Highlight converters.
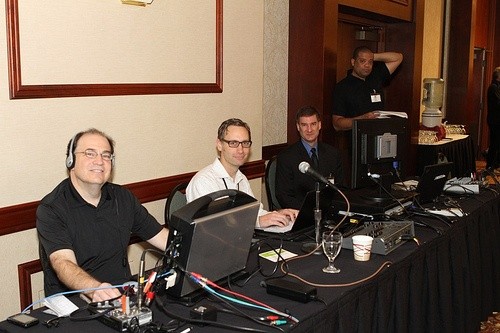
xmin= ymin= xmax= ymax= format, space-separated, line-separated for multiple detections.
xmin=266 ymin=279 xmax=318 ymax=304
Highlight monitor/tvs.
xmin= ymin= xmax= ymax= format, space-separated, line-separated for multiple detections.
xmin=351 ymin=118 xmax=407 ymax=202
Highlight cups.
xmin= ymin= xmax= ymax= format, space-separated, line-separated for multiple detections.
xmin=352 ymin=235 xmax=374 ymax=262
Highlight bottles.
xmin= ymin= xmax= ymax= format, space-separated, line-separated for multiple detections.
xmin=421 ymin=78 xmax=444 ymax=109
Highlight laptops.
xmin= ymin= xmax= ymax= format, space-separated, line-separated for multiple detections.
xmin=412 ymin=161 xmax=454 ymax=206
xmin=254 ymin=184 xmax=340 ymax=236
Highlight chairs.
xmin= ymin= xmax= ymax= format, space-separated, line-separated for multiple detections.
xmin=17 ymin=258 xmax=45 ymax=313
xmin=165 ymin=182 xmax=191 ymax=235
xmin=265 ymin=156 xmax=288 ymax=211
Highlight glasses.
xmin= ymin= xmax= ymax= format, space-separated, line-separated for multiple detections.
xmin=75 ymin=149 xmax=115 ymax=161
xmin=221 ymin=139 xmax=252 ymax=149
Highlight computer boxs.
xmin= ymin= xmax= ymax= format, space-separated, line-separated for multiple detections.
xmin=330 ymin=189 xmax=421 ymax=223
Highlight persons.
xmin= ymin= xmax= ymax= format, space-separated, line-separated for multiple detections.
xmin=36 ymin=128 xmax=169 ymax=304
xmin=185 ymin=117 xmax=299 ymax=228
xmin=487 ymin=67 xmax=500 ymax=170
xmin=275 ymin=106 xmax=354 ymax=225
xmin=331 ymin=47 xmax=403 ymax=184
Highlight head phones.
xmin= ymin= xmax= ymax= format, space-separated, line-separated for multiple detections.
xmin=66 ymin=136 xmax=77 ymax=169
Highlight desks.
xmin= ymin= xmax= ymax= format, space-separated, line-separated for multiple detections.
xmin=0 ymin=177 xmax=500 ymax=333
xmin=418 ymin=129 xmax=477 ymax=178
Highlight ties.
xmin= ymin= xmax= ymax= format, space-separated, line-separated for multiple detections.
xmin=310 ymin=148 xmax=320 ymax=174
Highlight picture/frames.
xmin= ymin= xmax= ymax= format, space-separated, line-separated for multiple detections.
xmin=5 ymin=0 xmax=225 ymax=100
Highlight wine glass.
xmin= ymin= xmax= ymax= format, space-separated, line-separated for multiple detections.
xmin=322 ymin=231 xmax=343 ymax=274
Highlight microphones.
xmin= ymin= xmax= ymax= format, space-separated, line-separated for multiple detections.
xmin=299 ymin=162 xmax=338 ymax=190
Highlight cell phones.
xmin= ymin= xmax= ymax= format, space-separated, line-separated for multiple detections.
xmin=7 ymin=313 xmax=39 ymax=328
